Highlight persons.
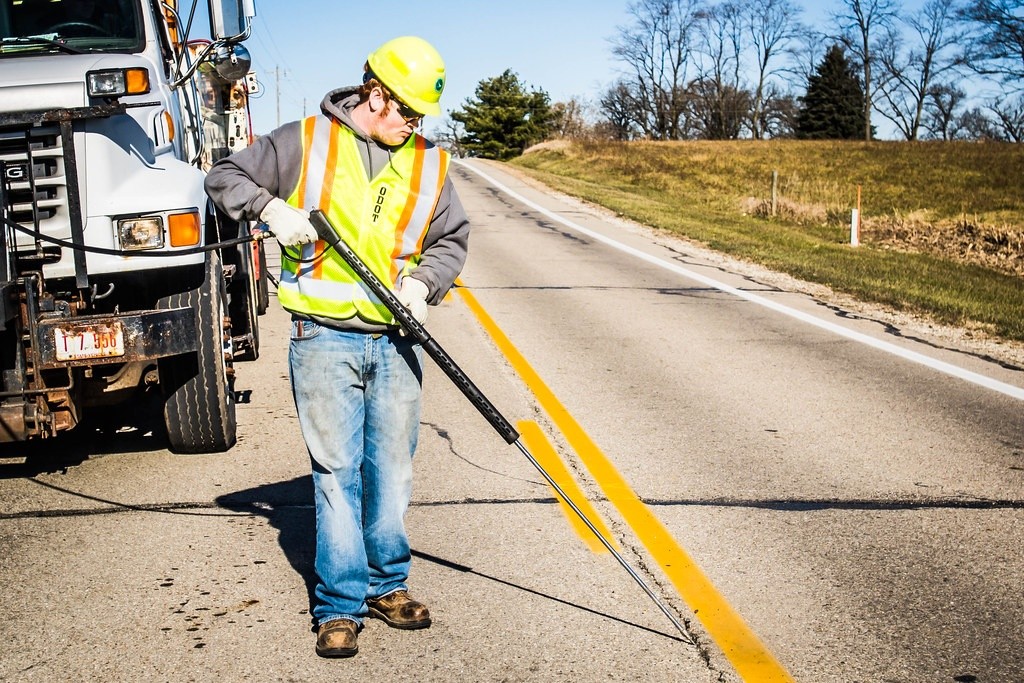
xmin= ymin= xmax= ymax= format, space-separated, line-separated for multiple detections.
xmin=200 ymin=34 xmax=477 ymax=660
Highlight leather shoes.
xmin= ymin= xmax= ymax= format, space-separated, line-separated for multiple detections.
xmin=315 ymin=619 xmax=360 ymax=658
xmin=366 ymin=591 xmax=432 ymax=629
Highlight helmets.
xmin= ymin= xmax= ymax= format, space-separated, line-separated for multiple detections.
xmin=366 ymin=36 xmax=446 ymax=115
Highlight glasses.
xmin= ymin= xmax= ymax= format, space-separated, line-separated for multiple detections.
xmin=388 ymin=93 xmax=426 ymax=123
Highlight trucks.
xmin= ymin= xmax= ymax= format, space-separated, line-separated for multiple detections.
xmin=1 ymin=0 xmax=271 ymax=453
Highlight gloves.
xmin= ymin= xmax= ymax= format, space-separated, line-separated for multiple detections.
xmin=389 ymin=279 xmax=430 ymax=337
xmin=259 ymin=196 xmax=319 ymax=247
